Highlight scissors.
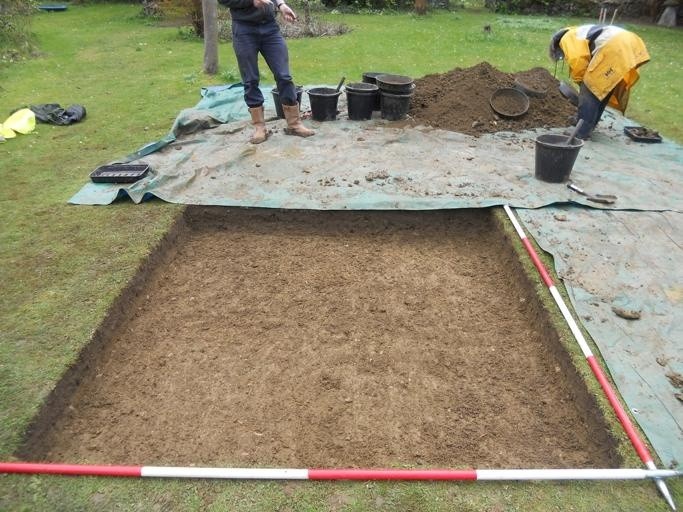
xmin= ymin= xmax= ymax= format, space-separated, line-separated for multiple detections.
xmin=567 ymin=183 xmax=617 ymax=203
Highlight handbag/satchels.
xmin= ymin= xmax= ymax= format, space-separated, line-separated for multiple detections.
xmin=277 ymin=3 xmax=286 ymax=8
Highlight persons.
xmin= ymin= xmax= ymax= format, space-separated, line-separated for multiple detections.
xmin=218 ymin=0 xmax=316 ymax=144
xmin=548 ymin=25 xmax=650 ymax=140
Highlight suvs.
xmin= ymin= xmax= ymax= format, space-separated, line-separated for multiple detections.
xmin=535 ymin=134 xmax=585 ymax=183
xmin=376 ymin=73 xmax=417 ymax=119
xmin=363 ymin=68 xmax=383 ymax=82
xmin=308 ymin=86 xmax=342 ymax=119
xmin=347 ymin=81 xmax=377 ymax=94
xmin=345 ymin=91 xmax=378 ymax=119
xmin=272 ymin=85 xmax=302 ymax=118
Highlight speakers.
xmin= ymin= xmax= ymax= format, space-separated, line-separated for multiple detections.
xmin=248 ymin=107 xmax=267 ymax=143
xmin=282 ymin=102 xmax=314 ymax=136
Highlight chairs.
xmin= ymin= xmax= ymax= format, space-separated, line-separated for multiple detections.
xmin=563 ymin=126 xmax=593 ymax=141
